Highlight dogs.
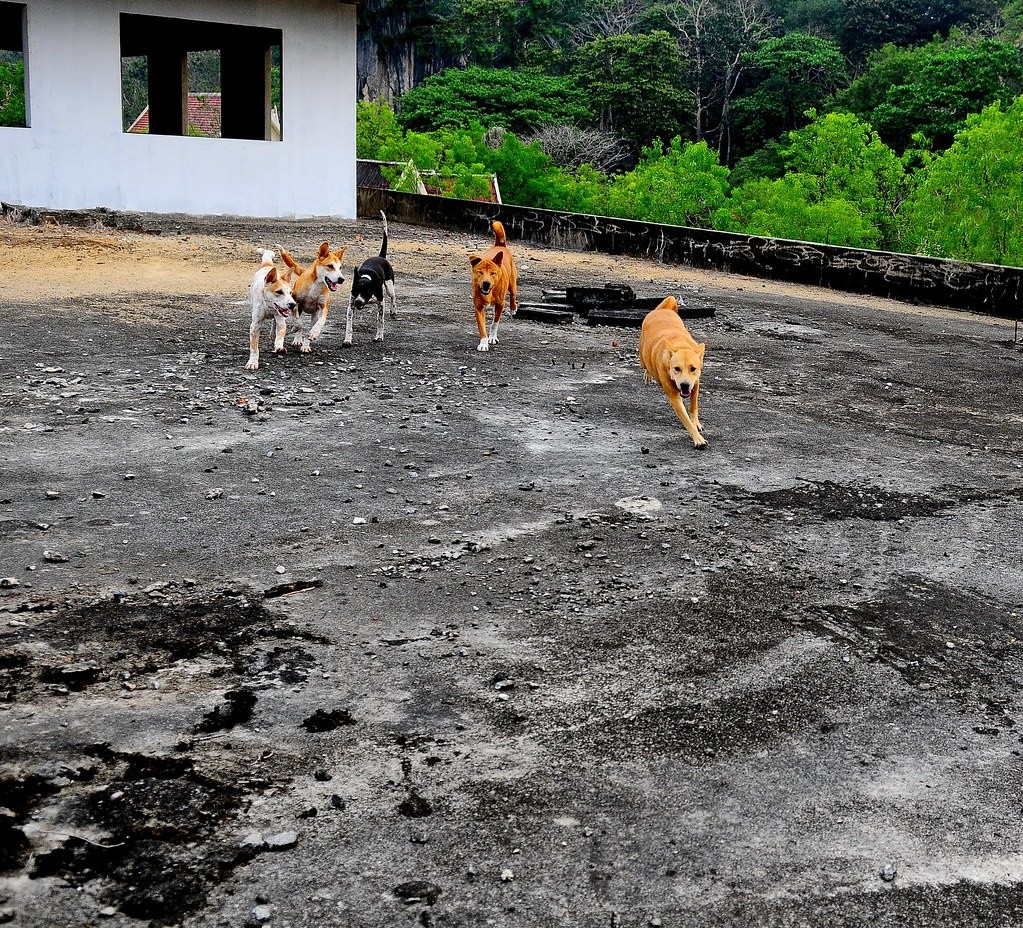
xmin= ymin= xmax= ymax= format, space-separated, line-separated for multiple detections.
xmin=640 ymin=296 xmax=709 ymax=449
xmin=244 ymin=210 xmax=396 ymax=370
xmin=469 ymin=221 xmax=518 ymax=352
